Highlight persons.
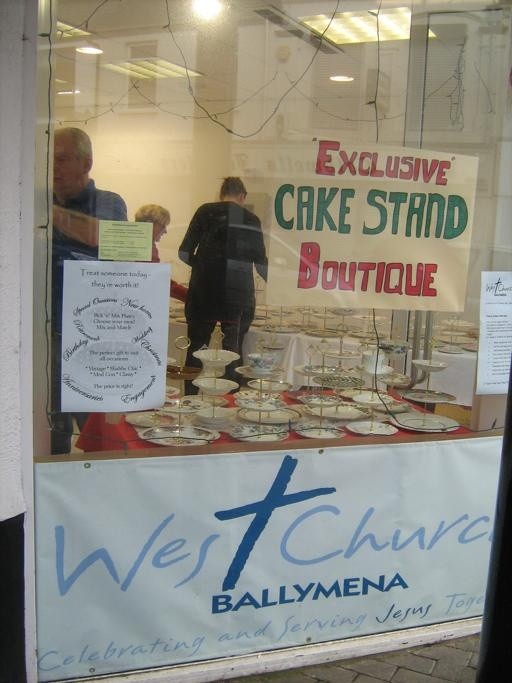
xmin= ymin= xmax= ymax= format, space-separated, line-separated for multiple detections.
xmin=133 ymin=204 xmax=188 ymax=304
xmin=177 ymin=178 xmax=268 ymax=400
xmin=52 ymin=126 xmax=130 ymax=455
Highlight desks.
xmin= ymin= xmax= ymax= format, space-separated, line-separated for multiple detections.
xmin=77 ymin=386 xmax=472 ymax=460
xmin=167 ymin=297 xmax=482 ymax=409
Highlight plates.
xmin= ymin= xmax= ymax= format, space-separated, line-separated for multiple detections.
xmin=125 ymin=289 xmax=478 ymax=446
